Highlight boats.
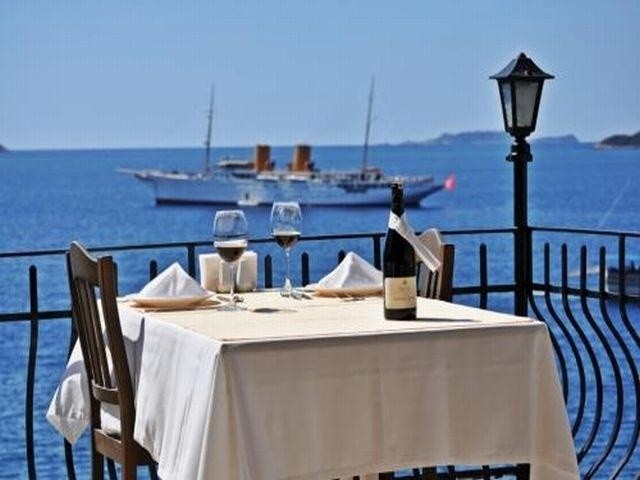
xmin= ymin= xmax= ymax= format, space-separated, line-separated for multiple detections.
xmin=606 ymin=267 xmax=638 ymax=296
xmin=114 ymin=76 xmax=454 ymax=210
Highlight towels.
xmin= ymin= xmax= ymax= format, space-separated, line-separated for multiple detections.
xmin=136 ymin=263 xmax=211 ymax=298
xmin=312 ymin=253 xmax=385 ymax=288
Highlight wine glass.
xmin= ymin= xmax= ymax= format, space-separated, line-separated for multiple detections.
xmin=268 ymin=199 xmax=304 ymax=294
xmin=211 ymin=210 xmax=250 ymax=313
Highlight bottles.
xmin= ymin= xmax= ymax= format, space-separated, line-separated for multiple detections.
xmin=381 ymin=181 xmax=419 ymax=320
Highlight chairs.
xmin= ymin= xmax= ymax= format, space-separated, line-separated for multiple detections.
xmin=415 ymin=229 xmax=454 ymax=304
xmin=66 ymin=242 xmax=160 ymax=480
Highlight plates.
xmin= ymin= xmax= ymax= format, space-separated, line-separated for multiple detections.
xmin=129 ymin=294 xmax=209 ymax=310
xmin=312 ymin=283 xmax=383 ymax=298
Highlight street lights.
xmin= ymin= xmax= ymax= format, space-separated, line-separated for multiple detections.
xmin=486 ymin=50 xmax=555 ymax=316
